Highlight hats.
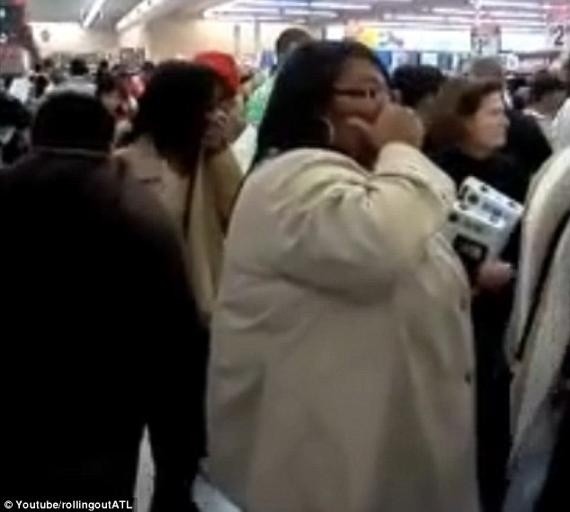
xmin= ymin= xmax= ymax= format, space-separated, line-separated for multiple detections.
xmin=194 ymin=52 xmax=240 ymax=97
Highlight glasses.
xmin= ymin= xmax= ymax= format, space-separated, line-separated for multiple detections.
xmin=336 ymin=86 xmax=403 ymax=102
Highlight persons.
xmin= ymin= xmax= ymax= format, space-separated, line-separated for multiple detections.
xmin=2 ymin=27 xmax=570 ymax=512
